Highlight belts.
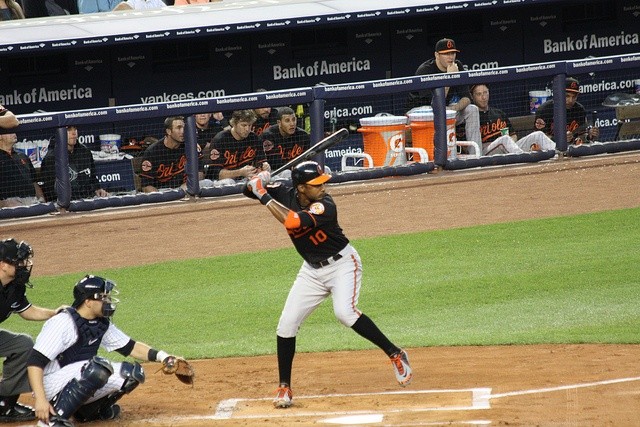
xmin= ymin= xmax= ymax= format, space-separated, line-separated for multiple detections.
xmin=307 ymin=250 xmax=347 ymax=268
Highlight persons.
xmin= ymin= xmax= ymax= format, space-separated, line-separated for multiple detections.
xmin=405 ymin=37 xmax=483 ymax=155
xmin=0 ymin=237 xmax=70 ymax=427
xmin=243 ymin=159 xmax=414 ymax=409
xmin=457 ymin=82 xmax=556 ymax=156
xmin=19 ymin=0 xmax=78 ymax=18
xmin=78 ymin=0 xmax=134 ymax=14
xmin=26 ymin=274 xmax=176 ymax=427
xmin=141 ymin=116 xmax=206 ymax=191
xmin=250 ymin=88 xmax=278 ymax=134
xmin=208 ymin=106 xmax=270 ymax=182
xmin=1 ymin=0 xmax=24 ymax=20
xmin=194 ymin=111 xmax=231 ymax=151
xmin=534 ymin=78 xmax=599 ymax=147
xmin=261 ymin=108 xmax=312 ymax=173
xmin=42 ymin=125 xmax=110 ymax=203
xmin=1 ymin=106 xmax=21 ymax=129
xmin=1 ymin=133 xmax=45 ymax=207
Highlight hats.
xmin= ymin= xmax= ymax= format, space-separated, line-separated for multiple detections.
xmin=565 ymin=76 xmax=579 ymax=93
xmin=290 ymin=161 xmax=332 ymax=185
xmin=435 ymin=37 xmax=461 ymax=54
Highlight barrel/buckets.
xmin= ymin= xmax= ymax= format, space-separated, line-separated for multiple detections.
xmin=32 ymin=139 xmax=52 ymax=164
xmin=407 ymin=105 xmax=458 ymax=160
xmin=13 ymin=140 xmax=38 ymax=168
xmin=99 ymin=132 xmax=121 ymax=154
xmin=358 ymin=112 xmax=408 ymax=167
xmin=528 ymin=91 xmax=549 ymax=115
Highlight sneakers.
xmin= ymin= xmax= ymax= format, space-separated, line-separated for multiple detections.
xmin=391 ymin=346 xmax=412 ymax=386
xmin=273 ymin=381 xmax=294 ymax=406
xmin=573 ymin=138 xmax=583 ymax=145
xmin=77 ymin=404 xmax=119 ymax=420
xmin=36 ymin=415 xmax=70 ymax=426
xmin=0 ymin=398 xmax=37 ymax=421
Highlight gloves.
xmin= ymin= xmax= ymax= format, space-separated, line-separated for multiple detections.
xmin=257 ymin=170 xmax=271 ymax=184
xmin=247 ymin=174 xmax=267 ymax=198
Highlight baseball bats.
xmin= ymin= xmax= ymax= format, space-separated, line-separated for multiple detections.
xmin=591 ymin=110 xmax=597 ymax=127
xmin=248 ymin=128 xmax=348 ymax=191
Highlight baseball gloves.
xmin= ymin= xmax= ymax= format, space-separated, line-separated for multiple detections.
xmin=158 ymin=357 xmax=193 ymax=387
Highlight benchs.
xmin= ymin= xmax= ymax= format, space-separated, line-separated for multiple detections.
xmin=132 ymin=150 xmax=212 ymax=190
xmin=509 ymin=104 xmax=636 ymax=140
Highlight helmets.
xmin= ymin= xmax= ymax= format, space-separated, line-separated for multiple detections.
xmin=74 ymin=275 xmax=119 ymax=316
xmin=1 ymin=237 xmax=32 ymax=284
